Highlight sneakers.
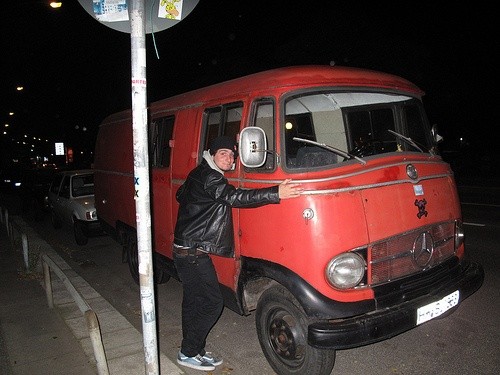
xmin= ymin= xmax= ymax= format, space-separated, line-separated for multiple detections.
xmin=202 ymin=351 xmax=223 ymax=366
xmin=177 ymin=351 xmax=216 ymax=371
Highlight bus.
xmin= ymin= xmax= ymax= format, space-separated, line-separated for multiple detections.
xmin=93 ymin=63 xmax=486 ymax=375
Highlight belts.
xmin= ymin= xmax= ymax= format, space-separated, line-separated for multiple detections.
xmin=173 ymin=246 xmax=206 ymax=257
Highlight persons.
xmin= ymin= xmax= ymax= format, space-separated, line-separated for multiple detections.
xmin=16 ymin=160 xmax=33 ymax=221
xmin=172 ymin=135 xmax=305 ymax=370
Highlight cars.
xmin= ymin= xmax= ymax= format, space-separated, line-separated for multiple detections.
xmin=47 ymin=170 xmax=103 ymax=245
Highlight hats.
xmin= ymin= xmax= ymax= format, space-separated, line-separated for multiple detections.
xmin=210 ymin=137 xmax=238 ymax=158
